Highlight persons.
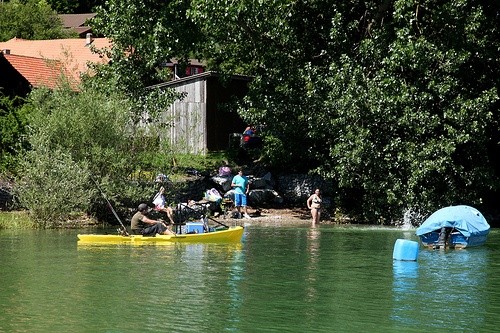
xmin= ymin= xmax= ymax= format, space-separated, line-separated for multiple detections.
xmin=230 ymin=169 xmax=251 ymax=220
xmin=307 ymin=188 xmax=323 ymax=225
xmin=130 ymin=204 xmax=175 ymax=236
xmin=152 ymin=186 xmax=175 ymax=225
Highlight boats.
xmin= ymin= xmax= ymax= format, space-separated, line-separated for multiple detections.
xmin=73 ymin=223 xmax=247 ymax=246
xmin=416 ymin=204 xmax=491 ymax=248
